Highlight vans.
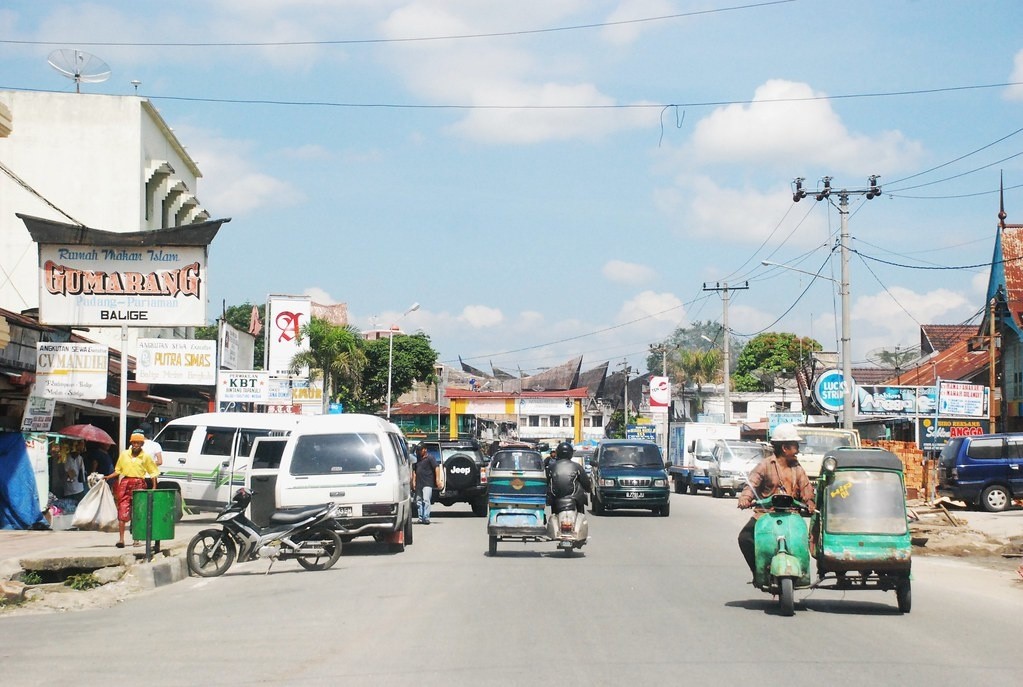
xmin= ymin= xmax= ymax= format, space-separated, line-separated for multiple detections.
xmin=151 ymin=411 xmax=306 ymax=513
xmin=708 ymin=439 xmax=768 ymax=498
xmin=573 ymin=446 xmax=597 ymax=483
xmin=244 ymin=413 xmax=419 ymax=552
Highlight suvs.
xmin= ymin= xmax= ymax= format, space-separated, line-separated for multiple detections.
xmin=409 ymin=438 xmax=490 ymax=516
xmin=590 ymin=438 xmax=673 ymax=516
xmin=936 ymin=432 xmax=1023 ymax=512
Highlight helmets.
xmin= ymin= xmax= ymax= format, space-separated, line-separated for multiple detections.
xmin=556 ymin=442 xmax=573 ymax=459
xmin=550 ymin=448 xmax=556 ymax=454
xmin=770 ymin=424 xmax=802 ymax=441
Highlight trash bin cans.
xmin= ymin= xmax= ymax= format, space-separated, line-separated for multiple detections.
xmin=132 ymin=489 xmax=177 ymax=540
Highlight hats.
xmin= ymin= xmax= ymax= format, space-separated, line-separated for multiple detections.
xmin=415 ymin=441 xmax=427 ymax=449
xmin=129 ymin=429 xmax=145 ymax=443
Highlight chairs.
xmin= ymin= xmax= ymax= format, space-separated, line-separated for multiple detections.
xmin=604 ymin=450 xmax=618 ymax=464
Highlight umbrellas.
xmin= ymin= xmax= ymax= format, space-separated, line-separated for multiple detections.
xmin=58 ymin=423 xmax=116 ymax=445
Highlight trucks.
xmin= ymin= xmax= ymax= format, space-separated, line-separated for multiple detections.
xmin=666 ymin=421 xmax=741 ymax=494
xmin=762 ymin=426 xmax=863 ymax=496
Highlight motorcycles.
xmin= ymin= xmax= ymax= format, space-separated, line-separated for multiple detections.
xmin=186 ymin=488 xmax=349 ymax=578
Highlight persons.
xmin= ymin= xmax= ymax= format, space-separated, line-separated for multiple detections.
xmin=544 ymin=448 xmax=557 ymax=471
xmin=46 ymin=422 xmax=162 ymax=548
xmin=738 ymin=422 xmax=817 ymax=589
xmin=544 ymin=442 xmax=594 ymax=514
xmin=411 ymin=441 xmax=442 ymax=524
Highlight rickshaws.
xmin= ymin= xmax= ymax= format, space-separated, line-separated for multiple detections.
xmin=739 ymin=445 xmax=912 ymax=616
xmin=486 ymin=450 xmax=589 ymax=556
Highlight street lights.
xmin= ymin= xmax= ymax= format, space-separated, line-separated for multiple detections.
xmin=701 ymin=335 xmax=730 ymax=421
xmin=761 ymin=260 xmax=853 ymax=433
xmin=387 ymin=302 xmax=419 ymax=423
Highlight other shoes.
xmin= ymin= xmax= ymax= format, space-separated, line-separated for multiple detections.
xmin=133 ymin=541 xmax=140 ymax=547
xmin=116 ymin=543 xmax=124 ymax=548
xmin=414 ymin=519 xmax=422 ymax=523
xmin=423 ymin=520 xmax=429 ymax=524
